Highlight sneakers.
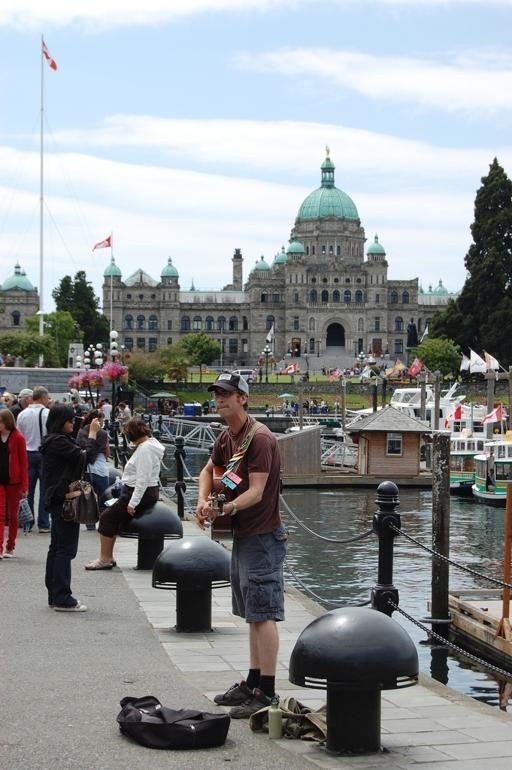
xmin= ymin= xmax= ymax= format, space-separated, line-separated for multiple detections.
xmin=52 ymin=603 xmax=88 ymax=612
xmin=214 ymin=681 xmax=252 ymax=705
xmin=48 ymin=599 xmax=81 ymax=608
xmin=39 ymin=527 xmax=51 ymax=533
xmin=230 ymin=686 xmax=279 ymax=719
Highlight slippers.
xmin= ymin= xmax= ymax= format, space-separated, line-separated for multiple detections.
xmin=88 ymin=558 xmax=117 ymax=566
xmin=85 ymin=559 xmax=113 ymax=570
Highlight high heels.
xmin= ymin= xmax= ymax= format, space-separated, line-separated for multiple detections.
xmin=3 ymin=544 xmax=14 ymax=558
xmin=0 ymin=549 xmax=4 ymax=559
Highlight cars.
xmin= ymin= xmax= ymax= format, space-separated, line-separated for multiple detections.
xmin=229 ymin=369 xmax=257 ymax=383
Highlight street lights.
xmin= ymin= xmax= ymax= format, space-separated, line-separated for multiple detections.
xmin=74 ymin=329 xmax=124 ymax=436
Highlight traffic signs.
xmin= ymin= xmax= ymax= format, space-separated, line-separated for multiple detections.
xmin=357 ymin=351 xmax=366 ymax=379
xmin=264 ymin=345 xmax=271 ymax=381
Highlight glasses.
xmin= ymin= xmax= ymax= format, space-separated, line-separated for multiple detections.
xmin=210 ymin=388 xmax=237 ymax=400
xmin=67 ymin=416 xmax=74 ymax=423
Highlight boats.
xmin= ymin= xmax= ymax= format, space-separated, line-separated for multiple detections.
xmin=349 ymin=382 xmax=512 ymax=502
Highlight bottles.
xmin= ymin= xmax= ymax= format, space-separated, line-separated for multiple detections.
xmin=114 ymin=476 xmax=121 ymax=489
xmin=268 ymin=697 xmax=282 ymax=738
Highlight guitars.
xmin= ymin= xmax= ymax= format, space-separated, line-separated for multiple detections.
xmin=202 ymin=466 xmax=233 ymax=539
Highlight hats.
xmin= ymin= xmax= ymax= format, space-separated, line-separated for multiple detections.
xmin=207 ymin=371 xmax=250 ymax=398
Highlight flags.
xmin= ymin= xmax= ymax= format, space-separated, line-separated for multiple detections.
xmin=93 ymin=236 xmax=111 ymax=250
xmin=444 ymin=405 xmax=461 ymax=424
xmin=481 ymin=405 xmax=502 ymax=425
xmin=326 ymin=349 xmax=501 ymax=385
xmin=42 ymin=39 xmax=58 ymax=70
xmin=265 ymin=325 xmax=274 ymax=343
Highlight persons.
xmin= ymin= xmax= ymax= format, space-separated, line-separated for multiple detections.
xmin=1 ymin=385 xmax=166 ymax=613
xmin=407 ymin=317 xmax=417 ymax=347
xmin=285 ymin=399 xmax=340 ymax=414
xmin=196 ymin=372 xmax=288 ymax=718
xmin=487 ymin=467 xmax=496 ymax=485
xmin=158 ymin=398 xmax=216 ymax=415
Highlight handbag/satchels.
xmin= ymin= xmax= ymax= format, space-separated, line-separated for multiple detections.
xmin=117 ymin=697 xmax=231 ymax=749
xmin=17 ymin=494 xmax=34 ymax=524
xmin=61 ymin=479 xmax=100 ymax=526
